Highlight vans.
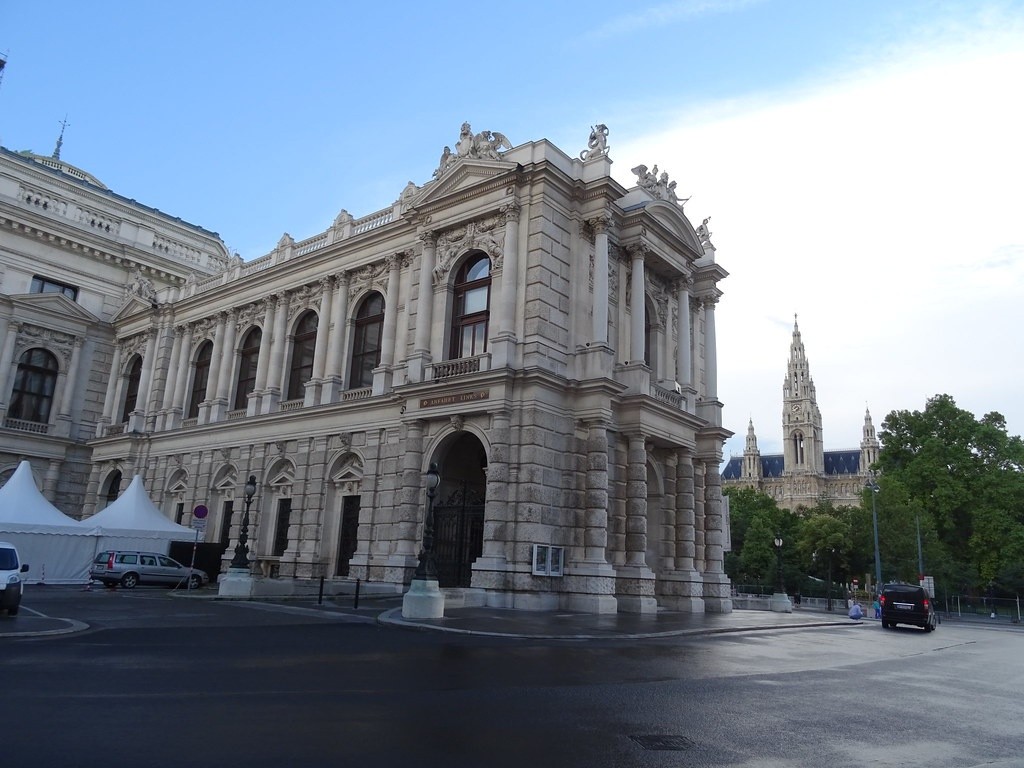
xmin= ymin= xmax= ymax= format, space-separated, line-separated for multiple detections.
xmin=89 ymin=550 xmax=209 ymax=590
xmin=881 ymin=584 xmax=936 ymax=633
xmin=0 ymin=542 xmax=30 ymax=616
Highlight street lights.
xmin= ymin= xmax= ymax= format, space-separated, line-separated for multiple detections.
xmin=866 ymin=470 xmax=882 ymax=595
xmin=230 ymin=475 xmax=258 ymax=570
xmin=775 ymin=533 xmax=783 ymax=593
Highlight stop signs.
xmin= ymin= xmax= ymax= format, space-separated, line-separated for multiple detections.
xmin=853 ymin=576 xmax=859 ymax=585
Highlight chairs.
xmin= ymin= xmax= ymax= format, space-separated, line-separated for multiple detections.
xmin=139 ymin=557 xmax=154 ymax=565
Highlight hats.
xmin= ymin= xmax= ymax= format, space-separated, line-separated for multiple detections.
xmin=859 ymin=602 xmax=864 ymax=607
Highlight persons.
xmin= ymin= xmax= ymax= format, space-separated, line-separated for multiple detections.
xmin=793 ymin=592 xmax=802 ymax=608
xmin=847 ymin=597 xmax=882 ymax=621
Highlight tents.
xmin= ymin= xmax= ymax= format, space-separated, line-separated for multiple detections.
xmin=0 ymin=460 xmax=207 ymax=586
